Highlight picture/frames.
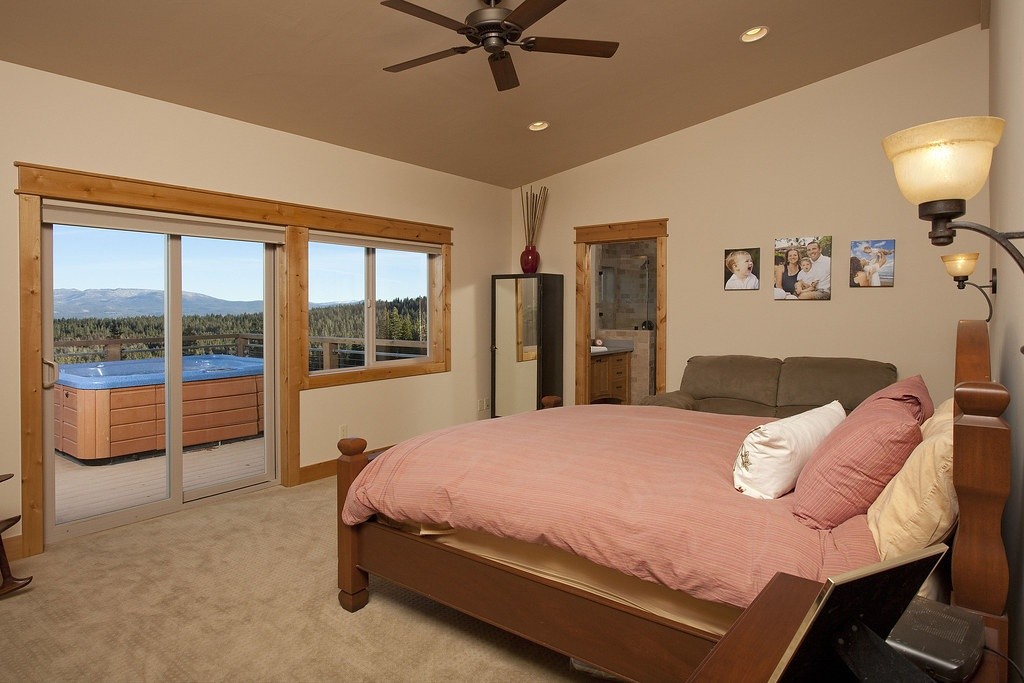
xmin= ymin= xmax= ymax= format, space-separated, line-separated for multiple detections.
xmin=772 ymin=543 xmax=949 ymax=683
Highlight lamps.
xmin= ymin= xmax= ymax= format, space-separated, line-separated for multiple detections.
xmin=880 ymin=114 xmax=1024 ymax=356
xmin=942 ymin=253 xmax=997 ymax=324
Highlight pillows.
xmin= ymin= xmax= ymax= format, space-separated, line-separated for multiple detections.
xmin=790 ymin=398 xmax=923 ymax=526
xmin=847 ymin=373 xmax=935 ymax=426
xmin=867 ymin=396 xmax=961 ymax=562
xmin=730 ymin=400 xmax=847 ymax=500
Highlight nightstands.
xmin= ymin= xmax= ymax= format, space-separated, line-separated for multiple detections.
xmin=684 ymin=572 xmax=1010 ymax=683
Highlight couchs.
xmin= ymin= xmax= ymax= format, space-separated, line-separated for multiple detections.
xmin=639 ymin=354 xmax=903 ymax=420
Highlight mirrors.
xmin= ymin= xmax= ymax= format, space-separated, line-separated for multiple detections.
xmin=495 ymin=277 xmax=538 ymax=417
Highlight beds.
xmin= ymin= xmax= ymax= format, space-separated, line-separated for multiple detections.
xmin=338 ymin=320 xmax=1013 ymax=683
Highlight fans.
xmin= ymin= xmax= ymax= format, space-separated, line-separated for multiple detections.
xmin=378 ymin=0 xmax=621 ymax=92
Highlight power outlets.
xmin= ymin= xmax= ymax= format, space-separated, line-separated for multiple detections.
xmin=478 ymin=400 xmax=484 ymax=411
xmin=484 ymin=398 xmax=490 ymax=410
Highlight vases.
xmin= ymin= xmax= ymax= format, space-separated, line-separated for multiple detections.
xmin=520 ymin=246 xmax=540 ymax=274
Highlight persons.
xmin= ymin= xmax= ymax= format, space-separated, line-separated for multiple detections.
xmin=859 ymin=250 xmax=886 ymax=286
xmin=794 ymin=241 xmax=831 ymax=299
xmin=851 ymin=257 xmax=876 ymax=287
xmin=863 ymin=246 xmax=894 ymax=256
xmin=725 ymin=251 xmax=759 ymax=289
xmin=774 ymin=249 xmax=802 ymax=298
xmin=794 ymin=257 xmax=819 ymax=294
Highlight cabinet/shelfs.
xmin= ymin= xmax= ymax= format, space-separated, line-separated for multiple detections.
xmin=591 ymin=351 xmax=631 ymax=406
xmin=490 ymin=273 xmax=563 ymax=421
